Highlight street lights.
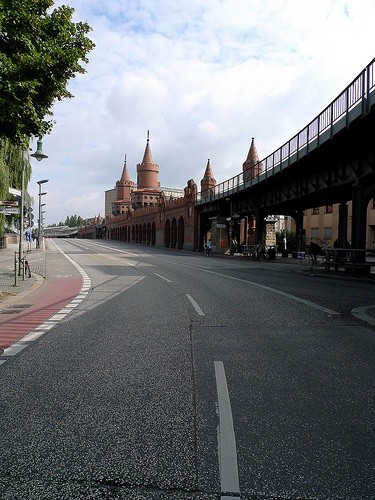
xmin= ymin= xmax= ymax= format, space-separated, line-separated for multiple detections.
xmin=18 ymin=137 xmax=50 ymax=275
xmin=245 ymin=216 xmax=248 ymax=245
xmin=36 ymin=178 xmax=49 ymax=248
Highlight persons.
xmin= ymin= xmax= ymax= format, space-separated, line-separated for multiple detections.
xmin=232 ymin=237 xmax=237 ymax=252
xmin=29 ymin=234 xmax=30 ymax=242
xmin=206 ymin=239 xmax=212 ymax=256
xmin=334 ymin=239 xmax=338 ymax=248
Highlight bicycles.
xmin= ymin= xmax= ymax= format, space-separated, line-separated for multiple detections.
xmin=15 ymin=250 xmax=33 ymax=278
